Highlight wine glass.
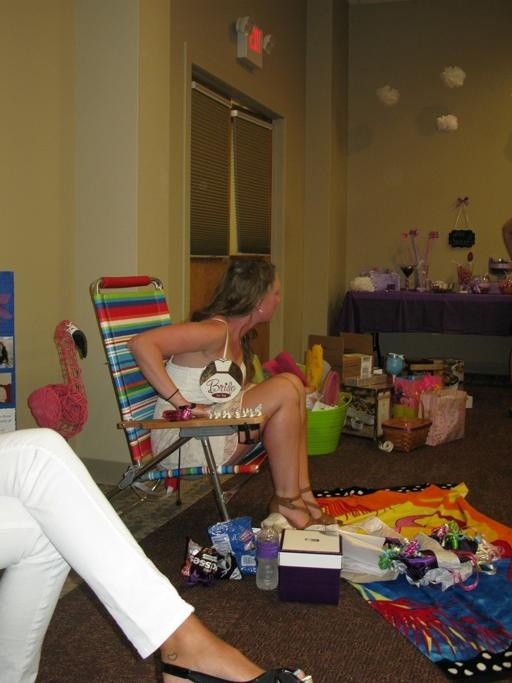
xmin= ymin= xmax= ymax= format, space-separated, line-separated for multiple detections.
xmin=398 ymin=263 xmax=417 ymax=293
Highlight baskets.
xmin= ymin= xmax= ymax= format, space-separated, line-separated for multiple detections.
xmin=382 ymin=416 xmax=432 ymax=452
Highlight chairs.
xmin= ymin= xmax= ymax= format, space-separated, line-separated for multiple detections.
xmin=92 ymin=275 xmax=266 ymax=523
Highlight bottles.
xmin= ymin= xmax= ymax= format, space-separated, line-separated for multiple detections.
xmin=255 ymin=519 xmax=281 ymax=591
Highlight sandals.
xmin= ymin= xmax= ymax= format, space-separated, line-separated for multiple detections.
xmin=269 ymin=487 xmax=342 ymax=530
xmin=158 ymin=660 xmax=313 ymax=682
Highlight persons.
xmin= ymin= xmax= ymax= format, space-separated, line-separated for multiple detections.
xmin=128 ymin=257 xmax=340 ymax=530
xmin=0 ymin=425 xmax=317 ymax=683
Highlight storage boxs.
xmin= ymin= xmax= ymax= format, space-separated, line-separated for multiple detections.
xmin=304 ymin=332 xmax=375 ymax=383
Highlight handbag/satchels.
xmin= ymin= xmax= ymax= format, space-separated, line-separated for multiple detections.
xmin=417 ymin=391 xmax=468 ymax=446
xmin=449 ymin=229 xmax=476 ymax=247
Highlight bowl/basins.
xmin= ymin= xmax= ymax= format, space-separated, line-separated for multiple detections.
xmin=431 ymin=280 xmax=455 ymax=292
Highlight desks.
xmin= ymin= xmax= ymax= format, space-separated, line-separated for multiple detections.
xmin=339 ymin=290 xmax=512 ymax=376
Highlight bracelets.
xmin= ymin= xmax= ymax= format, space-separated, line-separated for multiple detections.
xmin=166 ymin=388 xmax=180 ymax=402
